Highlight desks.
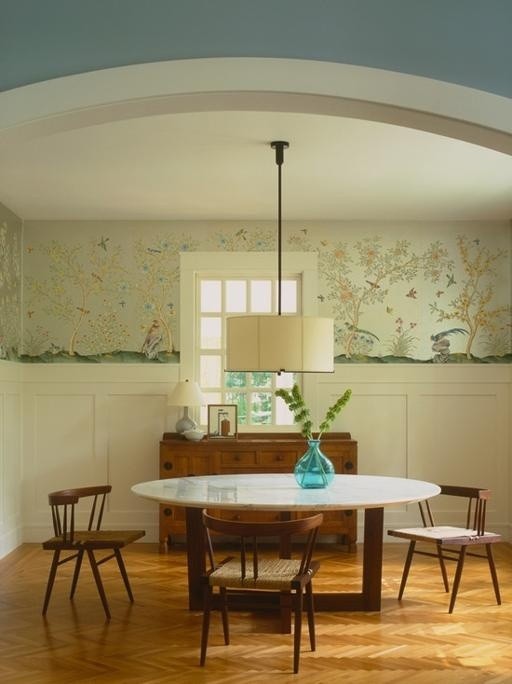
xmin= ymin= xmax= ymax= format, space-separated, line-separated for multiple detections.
xmin=132 ymin=471 xmax=443 ymax=612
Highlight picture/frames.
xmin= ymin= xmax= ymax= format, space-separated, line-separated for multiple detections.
xmin=207 ymin=404 xmax=239 ymax=441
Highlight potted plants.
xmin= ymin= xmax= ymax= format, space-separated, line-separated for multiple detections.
xmin=274 ymin=381 xmax=351 ymax=489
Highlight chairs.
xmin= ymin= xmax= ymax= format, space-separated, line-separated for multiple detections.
xmin=40 ymin=485 xmax=145 ymax=622
xmin=198 ymin=505 xmax=325 ymax=673
xmin=386 ymin=484 xmax=502 ymax=614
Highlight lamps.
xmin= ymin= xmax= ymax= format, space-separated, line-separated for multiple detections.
xmin=166 ymin=378 xmax=207 ymax=433
xmin=222 ymin=138 xmax=336 ymax=376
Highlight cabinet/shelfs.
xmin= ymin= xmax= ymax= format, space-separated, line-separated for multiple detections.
xmin=157 ymin=432 xmax=358 ymax=554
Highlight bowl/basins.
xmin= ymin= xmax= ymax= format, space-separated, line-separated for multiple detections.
xmin=183 ymin=430 xmax=204 ymax=442
